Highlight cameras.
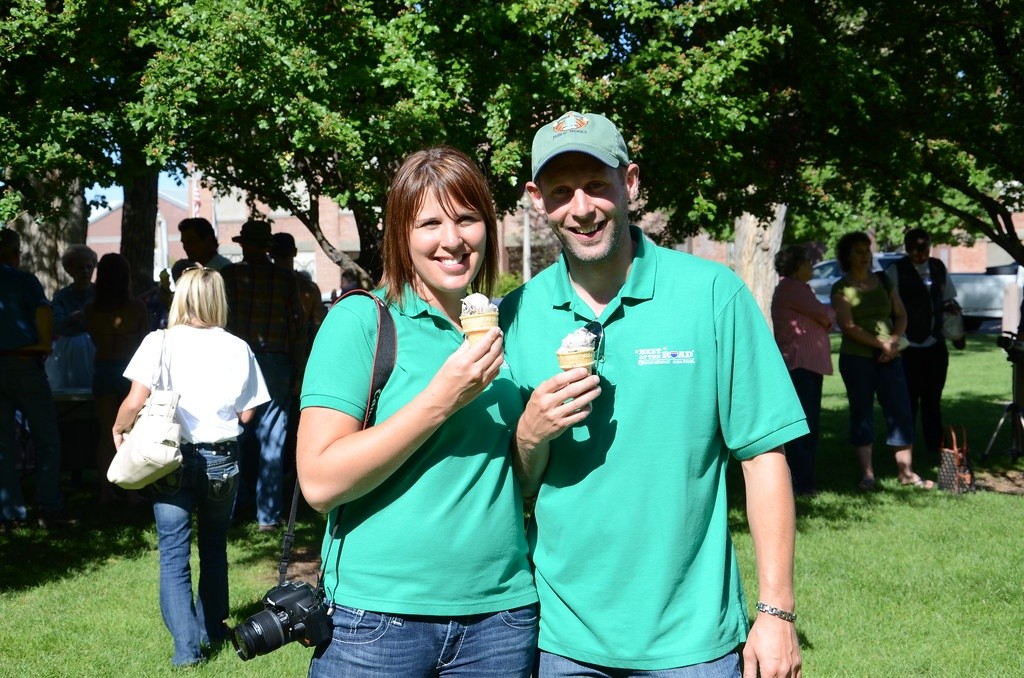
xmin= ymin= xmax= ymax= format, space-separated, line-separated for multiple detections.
xmin=230 ymin=580 xmax=335 ymax=661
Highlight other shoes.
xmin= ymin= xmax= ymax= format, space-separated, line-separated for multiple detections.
xmin=278 ymin=516 xmax=285 ymax=524
xmin=0 ymin=518 xmax=11 ymax=535
xmin=860 ymin=478 xmax=875 ymax=489
xmin=902 ymin=476 xmax=930 ymax=487
xmin=260 ymin=524 xmax=275 ymax=532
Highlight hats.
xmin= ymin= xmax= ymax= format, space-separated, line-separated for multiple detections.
xmin=232 ymin=220 xmax=272 ymax=245
xmin=532 ymin=111 xmax=628 ymax=183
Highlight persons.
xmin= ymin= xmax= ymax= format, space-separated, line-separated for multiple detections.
xmin=0 ymin=227 xmax=63 ymax=526
xmin=884 ymin=228 xmax=965 ymax=475
xmin=221 ymin=218 xmax=324 ymax=533
xmin=75 ymin=253 xmax=154 ymax=508
xmin=829 ymin=231 xmax=920 ymax=489
xmin=130 ymin=269 xmax=169 ymax=333
xmin=172 ymin=220 xmax=228 ymax=282
xmin=111 ymin=262 xmax=275 ymax=673
xmin=51 ymin=248 xmax=97 ymax=390
xmin=772 ymin=241 xmax=839 ymax=497
xmin=296 ymin=146 xmax=540 ymax=678
xmin=331 ymin=269 xmax=364 ymax=305
xmin=493 ymin=111 xmax=808 ymax=678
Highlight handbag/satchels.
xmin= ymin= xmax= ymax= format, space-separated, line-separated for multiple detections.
xmin=45 ymin=334 xmax=95 ymax=392
xmin=938 ymin=422 xmax=972 ymax=492
xmin=107 ymin=330 xmax=180 ymax=489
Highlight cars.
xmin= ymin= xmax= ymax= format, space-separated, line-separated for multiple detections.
xmin=806 ymin=252 xmax=906 ymax=332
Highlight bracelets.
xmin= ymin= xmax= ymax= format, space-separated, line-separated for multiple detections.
xmin=754 ymin=601 xmax=797 ymax=623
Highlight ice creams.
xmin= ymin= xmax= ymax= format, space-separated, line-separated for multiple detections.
xmin=459 ymin=292 xmax=499 ymax=347
xmin=556 ymin=327 xmax=596 ymax=375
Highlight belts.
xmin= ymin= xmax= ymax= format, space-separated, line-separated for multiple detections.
xmin=0 ymin=347 xmax=44 ymax=358
